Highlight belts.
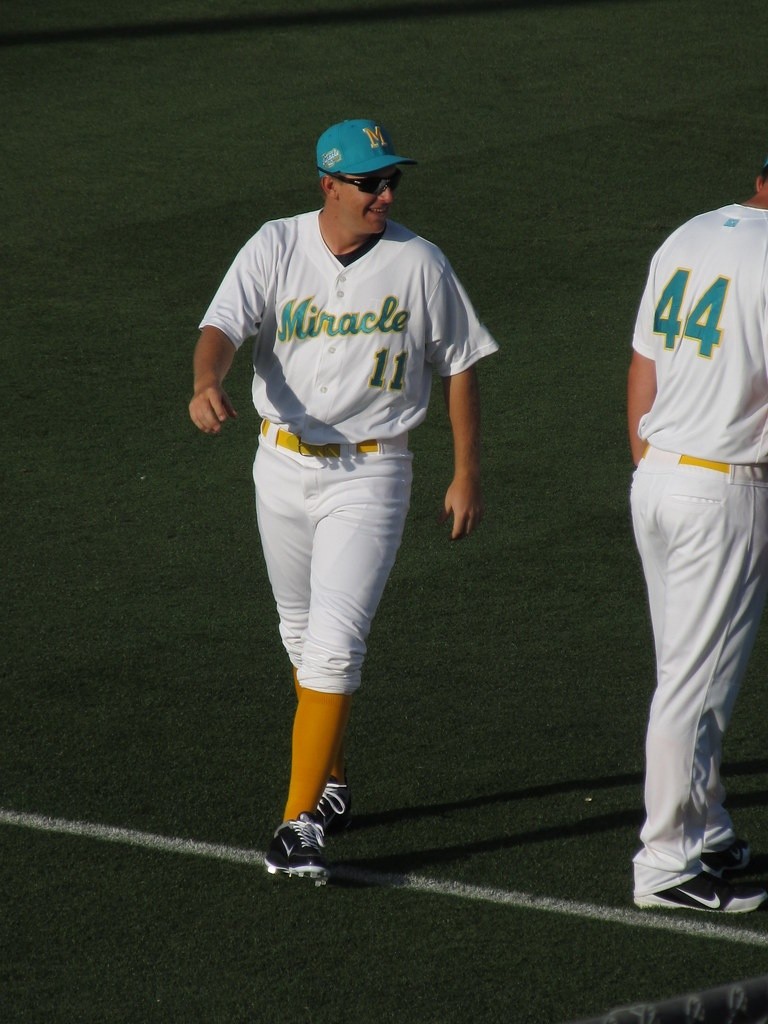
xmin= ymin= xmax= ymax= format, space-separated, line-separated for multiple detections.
xmin=261 ymin=419 xmax=379 ymax=458
xmin=641 ymin=443 xmax=731 ymax=473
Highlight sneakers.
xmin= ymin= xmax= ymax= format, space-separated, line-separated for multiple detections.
xmin=699 ymin=838 xmax=750 ymax=878
xmin=315 ymin=774 xmax=352 ymax=831
xmin=632 ymin=869 xmax=768 ymax=913
xmin=265 ymin=811 xmax=330 ymax=886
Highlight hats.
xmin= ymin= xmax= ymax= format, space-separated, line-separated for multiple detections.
xmin=316 ymin=119 xmax=417 ymax=178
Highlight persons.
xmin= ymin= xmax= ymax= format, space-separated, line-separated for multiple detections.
xmin=626 ymin=161 xmax=768 ymax=913
xmin=188 ymin=119 xmax=499 ymax=886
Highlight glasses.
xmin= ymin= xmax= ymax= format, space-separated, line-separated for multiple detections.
xmin=317 ymin=166 xmax=402 ymax=195
